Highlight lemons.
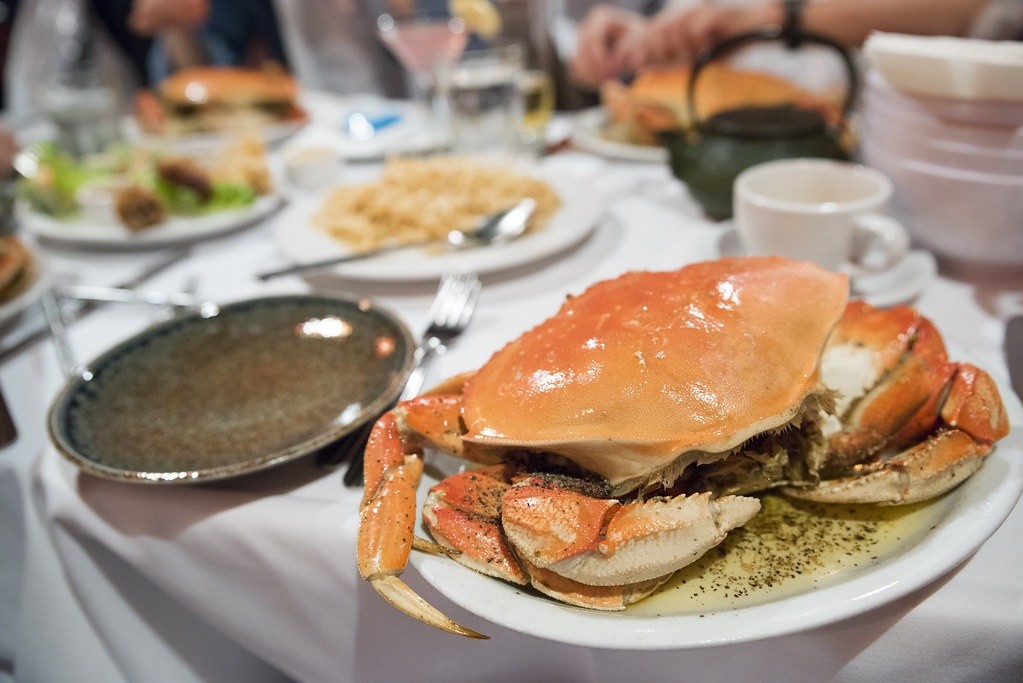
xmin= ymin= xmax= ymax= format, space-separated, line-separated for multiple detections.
xmin=446 ymin=0 xmax=503 ymax=38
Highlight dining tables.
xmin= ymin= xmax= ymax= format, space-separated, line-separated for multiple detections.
xmin=0 ymin=93 xmax=1023 ymax=682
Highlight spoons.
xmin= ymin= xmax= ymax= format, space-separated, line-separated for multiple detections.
xmin=252 ymin=194 xmax=537 ymax=283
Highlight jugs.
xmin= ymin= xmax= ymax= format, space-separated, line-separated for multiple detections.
xmin=651 ymin=31 xmax=853 ymax=219
xmin=272 ymin=1 xmax=415 ymax=108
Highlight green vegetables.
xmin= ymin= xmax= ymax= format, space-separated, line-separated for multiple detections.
xmin=13 ymin=137 xmax=258 ymax=219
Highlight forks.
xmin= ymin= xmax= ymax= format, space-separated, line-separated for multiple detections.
xmin=345 ymin=278 xmax=480 ymax=486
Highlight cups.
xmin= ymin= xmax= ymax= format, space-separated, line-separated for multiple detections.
xmin=1 ymin=3 xmax=140 ymax=168
xmin=732 ymin=157 xmax=909 ymax=282
xmin=436 ymin=42 xmax=558 ymax=167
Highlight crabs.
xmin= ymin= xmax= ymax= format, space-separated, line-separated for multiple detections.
xmin=602 ymin=59 xmax=863 ymax=161
xmin=139 ymin=67 xmax=306 ymax=148
xmin=357 ymin=256 xmax=1013 ymax=638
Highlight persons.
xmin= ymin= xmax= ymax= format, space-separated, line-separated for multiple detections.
xmin=0 ymin=0 xmax=1023 ymax=112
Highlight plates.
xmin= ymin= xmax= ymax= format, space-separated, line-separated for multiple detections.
xmin=282 ymin=171 xmax=602 ymax=286
xmin=46 ymin=293 xmax=416 ymax=485
xmin=851 ymin=248 xmax=937 ymax=307
xmin=17 ymin=196 xmax=282 ymax=251
xmin=569 ymin=110 xmax=674 ymax=163
xmin=405 ymin=358 xmax=1023 ymax=652
xmin=112 ymin=118 xmax=310 ymax=161
xmin=1 ymin=250 xmax=58 ymax=321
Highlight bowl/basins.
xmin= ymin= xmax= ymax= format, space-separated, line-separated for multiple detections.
xmin=853 ymin=34 xmax=1022 ymax=275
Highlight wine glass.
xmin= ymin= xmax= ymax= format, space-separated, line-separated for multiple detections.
xmin=377 ymin=15 xmax=470 ymax=147
